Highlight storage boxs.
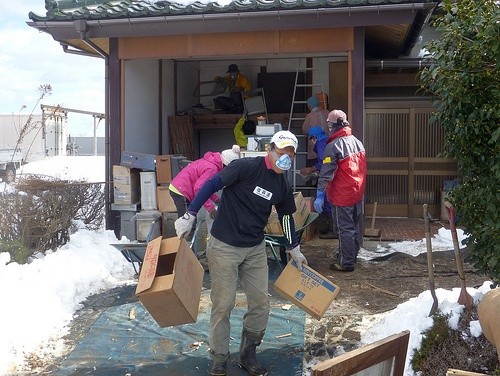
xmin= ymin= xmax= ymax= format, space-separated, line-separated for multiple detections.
xmin=113 ymin=165 xmax=139 ymax=205
xmin=135 ymin=236 xmax=204 ymax=328
xmin=265 ymin=192 xmax=308 ymax=235
xmin=274 ymin=257 xmax=340 ymax=321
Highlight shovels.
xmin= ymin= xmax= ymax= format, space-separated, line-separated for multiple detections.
xmin=444 ymin=201 xmax=474 ymax=307
xmin=364 ymin=202 xmax=380 ymax=236
xmin=422 ymin=204 xmax=438 ymax=317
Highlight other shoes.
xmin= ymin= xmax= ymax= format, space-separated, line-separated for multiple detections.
xmin=335 ymin=250 xmax=357 ymax=258
xmin=319 ymin=231 xmax=339 ymax=239
xmin=329 ymin=262 xmax=354 ymax=272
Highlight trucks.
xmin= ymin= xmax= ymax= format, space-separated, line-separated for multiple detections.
xmin=0 ymin=149 xmax=23 ymax=184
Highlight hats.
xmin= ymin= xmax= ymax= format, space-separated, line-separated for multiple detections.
xmin=308 ymin=126 xmax=325 ymax=140
xmin=327 ymin=109 xmax=347 ymax=123
xmin=271 ymin=130 xmax=298 ymax=153
xmin=226 ymin=64 xmax=240 ymax=73
xmin=307 ymin=95 xmax=320 ymax=108
xmin=221 ymin=145 xmax=240 ymax=166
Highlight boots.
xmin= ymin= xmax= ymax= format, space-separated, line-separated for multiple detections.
xmin=208 ymin=349 xmax=231 ymax=376
xmin=237 ymin=327 xmax=271 ymax=376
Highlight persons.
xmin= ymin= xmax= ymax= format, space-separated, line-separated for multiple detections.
xmin=168 ymin=145 xmax=241 ymax=243
xmin=174 ymin=131 xmax=309 ymax=376
xmin=314 ymin=109 xmax=366 ymax=271
xmin=302 ymin=96 xmax=331 ymax=186
xmin=216 ymin=64 xmax=253 ymax=114
xmin=300 ymin=126 xmax=340 ymax=239
xmin=234 ymin=110 xmax=256 ymax=150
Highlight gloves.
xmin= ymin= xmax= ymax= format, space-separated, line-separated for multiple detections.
xmin=313 ymin=189 xmax=325 ymax=214
xmin=286 ymin=243 xmax=308 ymax=271
xmin=175 ymin=212 xmax=196 ymax=240
